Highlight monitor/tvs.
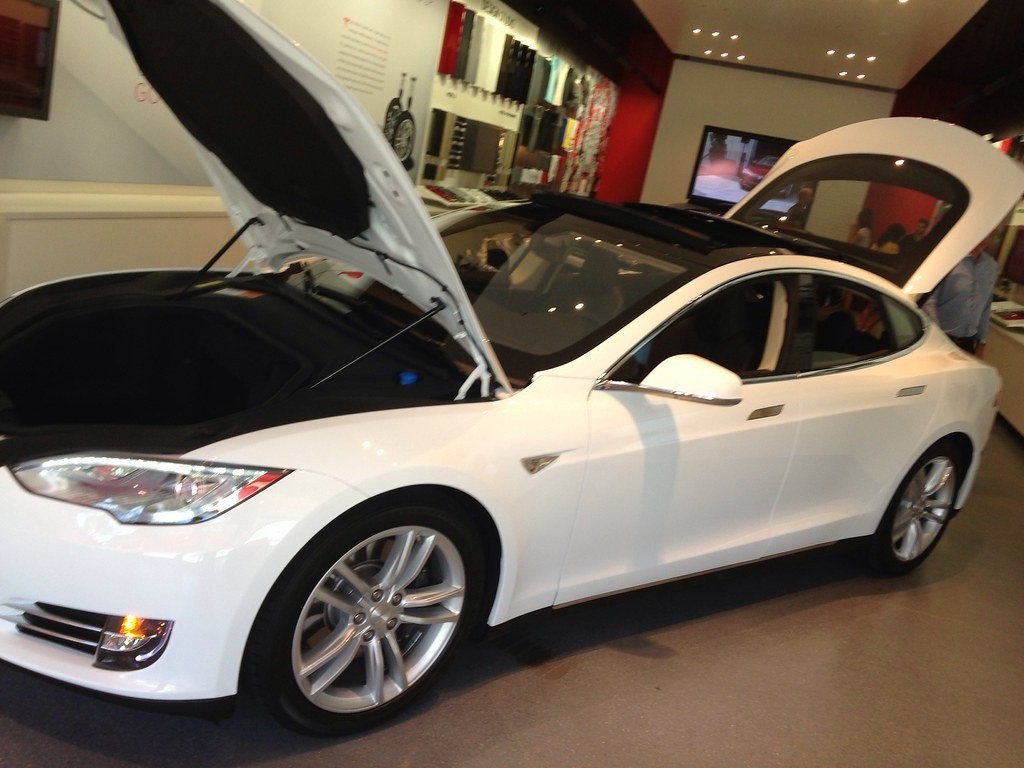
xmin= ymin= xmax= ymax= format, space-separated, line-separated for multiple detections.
xmin=685 ymin=125 xmax=820 ymax=231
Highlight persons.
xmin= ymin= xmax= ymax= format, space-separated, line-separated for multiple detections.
xmin=921 ymin=229 xmax=998 ymax=358
xmin=847 ymin=208 xmax=872 ymax=247
xmin=869 ymin=222 xmax=905 ymax=254
xmin=905 ymin=217 xmax=928 ymax=252
xmin=787 ymin=188 xmax=812 ymax=228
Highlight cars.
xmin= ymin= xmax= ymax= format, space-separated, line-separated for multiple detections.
xmin=0 ymin=0 xmax=1023 ymax=741
xmin=738 ymin=154 xmax=798 ymax=199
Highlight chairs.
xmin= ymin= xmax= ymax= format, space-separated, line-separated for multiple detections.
xmin=540 ymin=239 xmax=619 ymax=317
xmin=644 ymin=276 xmax=786 ymax=378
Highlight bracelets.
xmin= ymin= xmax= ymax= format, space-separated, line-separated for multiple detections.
xmin=977 ymin=339 xmax=986 ymax=345
